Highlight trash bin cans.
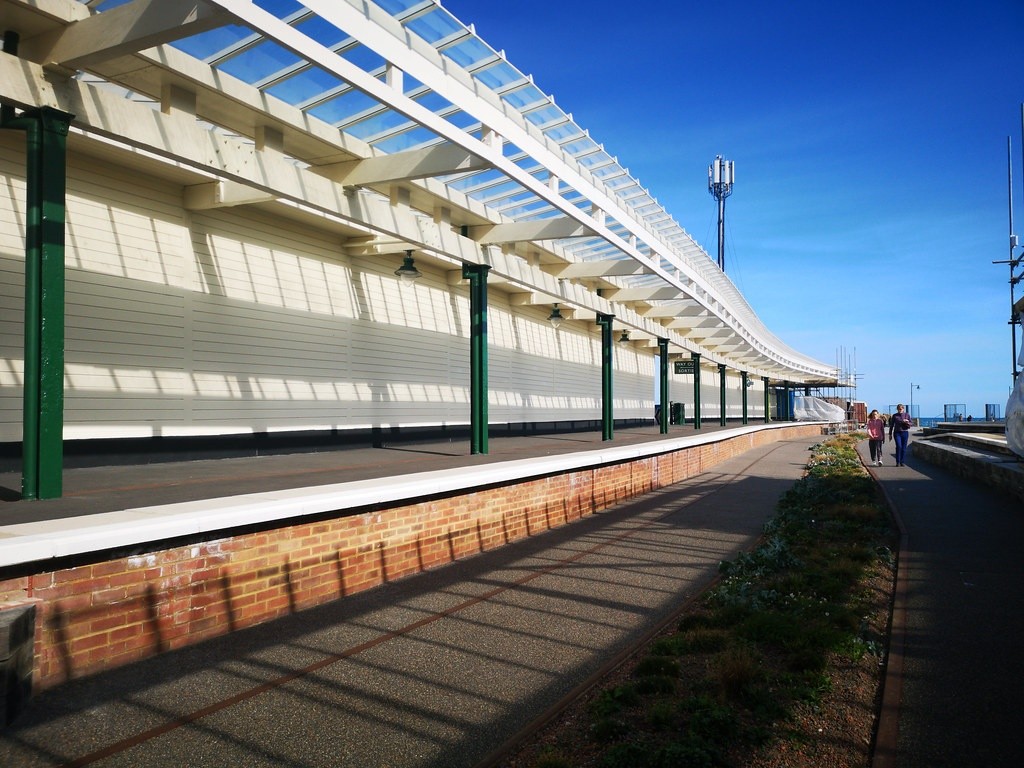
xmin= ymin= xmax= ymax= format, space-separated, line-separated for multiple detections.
xmin=672 ymin=403 xmax=685 ymax=425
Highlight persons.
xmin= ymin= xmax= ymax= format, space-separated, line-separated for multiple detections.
xmin=882 ymin=413 xmax=892 ymax=427
xmin=866 ymin=409 xmax=886 ymax=467
xmin=967 ymin=415 xmax=972 ymax=422
xmin=959 ymin=413 xmax=963 ymax=422
xmin=889 ymin=404 xmax=913 ymax=467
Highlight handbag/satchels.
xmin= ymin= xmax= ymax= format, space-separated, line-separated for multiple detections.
xmin=901 ymin=413 xmax=910 ymax=430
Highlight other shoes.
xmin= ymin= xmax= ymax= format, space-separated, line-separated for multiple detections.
xmin=872 ymin=461 xmax=876 ymax=466
xmin=900 ymin=463 xmax=905 ymax=467
xmin=896 ymin=463 xmax=900 ymax=467
xmin=878 ymin=461 xmax=883 ymax=467
